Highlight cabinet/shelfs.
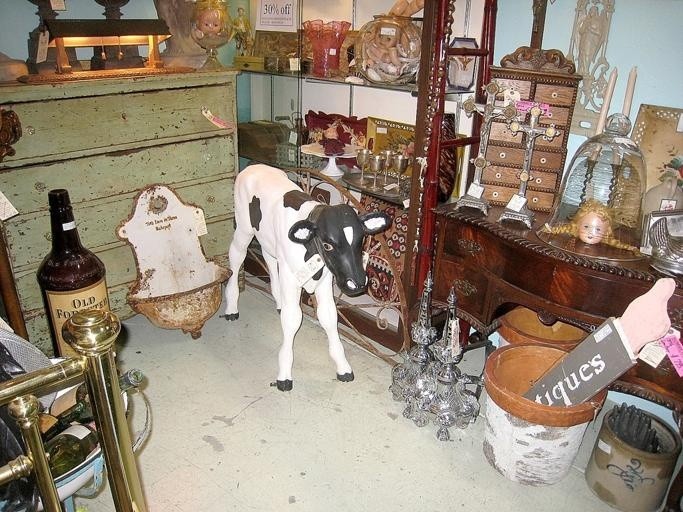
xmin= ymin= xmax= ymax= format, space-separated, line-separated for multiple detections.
xmin=0 ymin=72 xmax=243 ymax=358
xmin=476 ymin=46 xmax=583 ymax=215
xmin=234 ymin=0 xmax=496 ymax=351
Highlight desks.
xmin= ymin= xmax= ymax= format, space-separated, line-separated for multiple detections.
xmin=430 ymin=202 xmax=683 ymax=440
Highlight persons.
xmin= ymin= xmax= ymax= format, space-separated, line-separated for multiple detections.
xmin=229 ymin=7 xmax=251 ymax=51
xmin=193 ymin=10 xmax=225 ymax=41
xmin=361 ymin=27 xmax=407 ymax=71
xmin=538 ymin=200 xmax=643 ymax=258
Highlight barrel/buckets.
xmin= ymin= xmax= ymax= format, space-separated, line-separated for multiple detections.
xmin=481 ymin=342 xmax=608 ymax=488
xmin=497 ymin=307 xmax=589 ymax=349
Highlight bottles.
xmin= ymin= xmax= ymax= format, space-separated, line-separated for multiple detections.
xmin=353 ymin=15 xmax=422 ymax=87
xmin=35 ymin=188 xmax=117 ymax=371
xmin=36 ymin=368 xmax=144 ymax=480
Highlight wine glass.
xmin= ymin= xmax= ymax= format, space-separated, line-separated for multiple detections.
xmin=188 ymin=2 xmax=232 ymax=72
xmin=356 ymin=151 xmax=408 ymax=191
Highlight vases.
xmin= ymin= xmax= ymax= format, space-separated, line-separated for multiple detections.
xmin=302 ymin=20 xmax=351 ymax=78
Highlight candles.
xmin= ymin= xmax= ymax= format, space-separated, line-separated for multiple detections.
xmin=612 ymin=66 xmax=638 ymax=166
xmin=589 ymin=66 xmax=618 ymax=162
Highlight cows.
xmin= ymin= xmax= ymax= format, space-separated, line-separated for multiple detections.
xmin=224 ymin=163 xmax=392 ymax=391
